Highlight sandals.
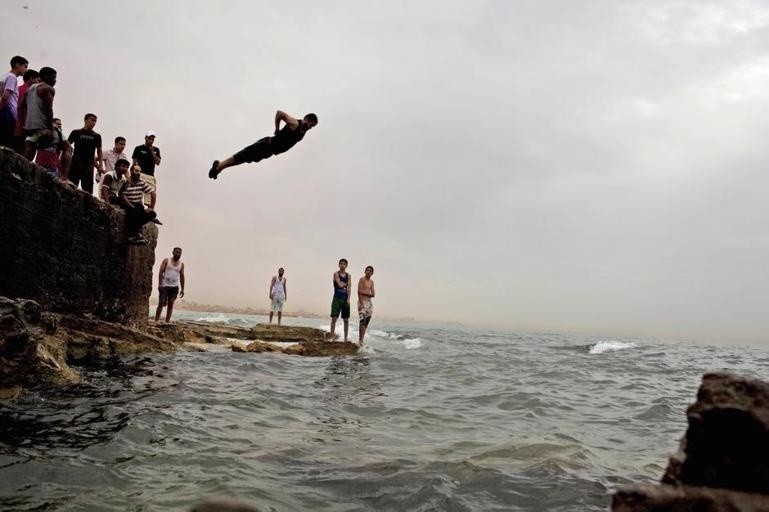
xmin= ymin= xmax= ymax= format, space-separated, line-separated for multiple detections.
xmin=125 ymin=238 xmax=144 ymax=244
xmin=136 ymin=233 xmax=150 ymax=246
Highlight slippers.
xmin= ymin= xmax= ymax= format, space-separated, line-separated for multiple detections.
xmin=209 ymin=161 xmax=219 ymax=178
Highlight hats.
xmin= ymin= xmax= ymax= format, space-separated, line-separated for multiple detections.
xmin=144 ymin=131 xmax=155 ymax=137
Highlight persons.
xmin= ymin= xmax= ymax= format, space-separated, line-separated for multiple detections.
xmin=328 ymin=258 xmax=353 ymax=342
xmin=0 ymin=56 xmax=164 ymax=246
xmin=208 ymin=109 xmax=319 ymax=181
xmin=154 ymin=246 xmax=185 ymax=323
xmin=269 ymin=266 xmax=287 ymax=325
xmin=356 ymin=264 xmax=377 ymax=346
